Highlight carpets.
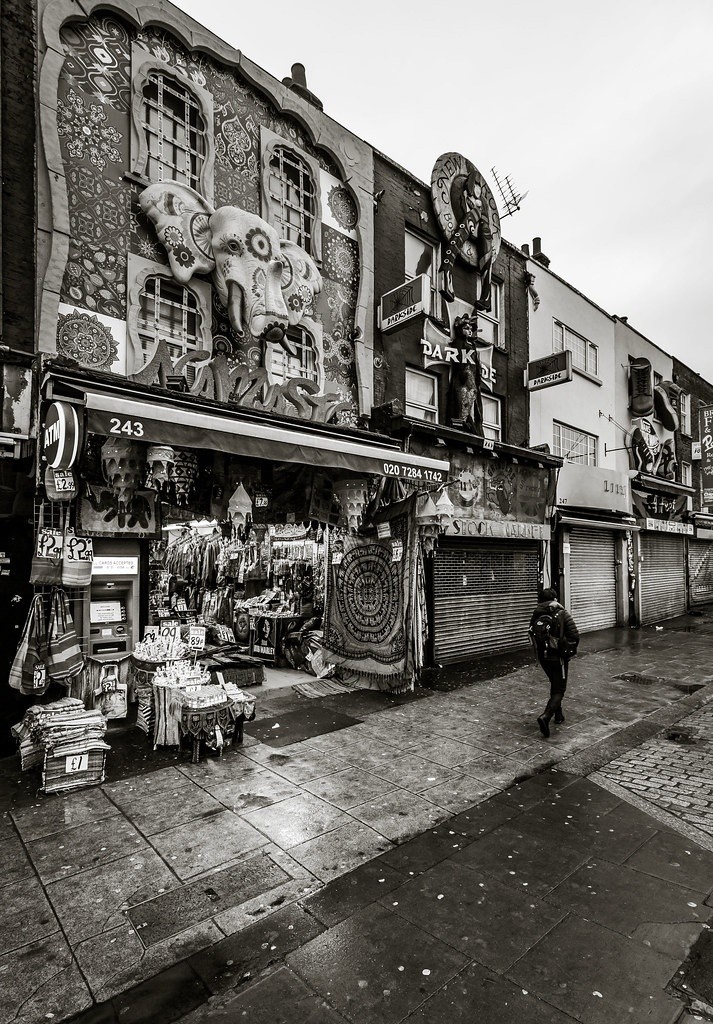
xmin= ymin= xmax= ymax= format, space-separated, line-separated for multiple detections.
xmin=292 ymin=678 xmax=358 ymax=699
xmin=243 ymin=706 xmax=364 ymax=748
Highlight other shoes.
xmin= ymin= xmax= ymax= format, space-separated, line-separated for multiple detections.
xmin=538 ymin=715 xmax=550 ymax=738
xmin=554 ymin=716 xmax=564 ymax=724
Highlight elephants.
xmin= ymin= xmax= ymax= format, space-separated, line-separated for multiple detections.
xmin=133 ymin=177 xmax=325 ymax=356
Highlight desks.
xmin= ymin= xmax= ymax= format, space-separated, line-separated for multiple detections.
xmin=179 ymin=698 xmax=234 ymax=762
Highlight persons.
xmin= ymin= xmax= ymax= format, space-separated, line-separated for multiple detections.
xmin=530 ymin=588 xmax=579 ymax=737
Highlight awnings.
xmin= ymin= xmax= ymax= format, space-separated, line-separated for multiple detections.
xmin=52 ymin=378 xmax=451 ymax=482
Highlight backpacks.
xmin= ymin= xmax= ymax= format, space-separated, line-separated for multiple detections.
xmin=529 ymin=603 xmax=563 ymax=662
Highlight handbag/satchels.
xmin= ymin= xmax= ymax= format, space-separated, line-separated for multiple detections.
xmin=9 ymin=465 xmax=93 ymax=696
